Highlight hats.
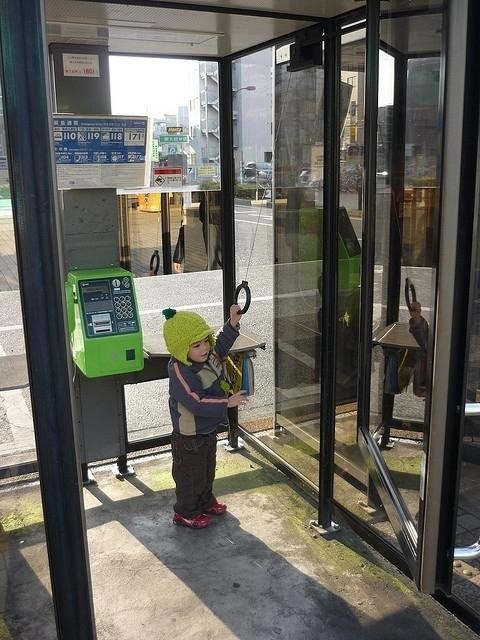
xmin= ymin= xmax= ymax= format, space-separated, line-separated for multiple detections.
xmin=163 ymin=309 xmax=214 ymax=366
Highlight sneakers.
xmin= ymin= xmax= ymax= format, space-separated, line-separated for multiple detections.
xmin=173 ymin=512 xmax=212 ymax=528
xmin=204 ymin=502 xmax=227 ymax=515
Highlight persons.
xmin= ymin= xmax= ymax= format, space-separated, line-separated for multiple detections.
xmin=408 ymin=300 xmax=428 ymax=349
xmin=163 ymin=303 xmax=249 ymax=529
xmin=172 ymin=216 xmax=185 ymax=273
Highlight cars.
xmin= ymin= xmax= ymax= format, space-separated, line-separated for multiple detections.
xmin=300 ymin=171 xmax=322 ymax=189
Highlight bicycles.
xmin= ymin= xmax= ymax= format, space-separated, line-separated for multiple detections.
xmin=340 ymin=169 xmax=361 ymax=193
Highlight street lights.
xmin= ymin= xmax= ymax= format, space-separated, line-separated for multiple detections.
xmin=232 ymin=85 xmax=256 ymax=99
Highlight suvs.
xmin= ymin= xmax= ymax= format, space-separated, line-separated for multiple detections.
xmin=244 ymin=162 xmax=273 ymax=182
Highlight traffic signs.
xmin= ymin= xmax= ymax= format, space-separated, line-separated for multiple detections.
xmin=158 ymin=135 xmax=189 ymax=143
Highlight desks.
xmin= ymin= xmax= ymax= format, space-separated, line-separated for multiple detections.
xmin=371 ymin=321 xmax=433 ymax=449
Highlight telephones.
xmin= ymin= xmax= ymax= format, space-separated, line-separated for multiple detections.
xmin=64 ymin=266 xmax=145 ymax=378
xmin=298 ymin=205 xmax=363 ymax=296
